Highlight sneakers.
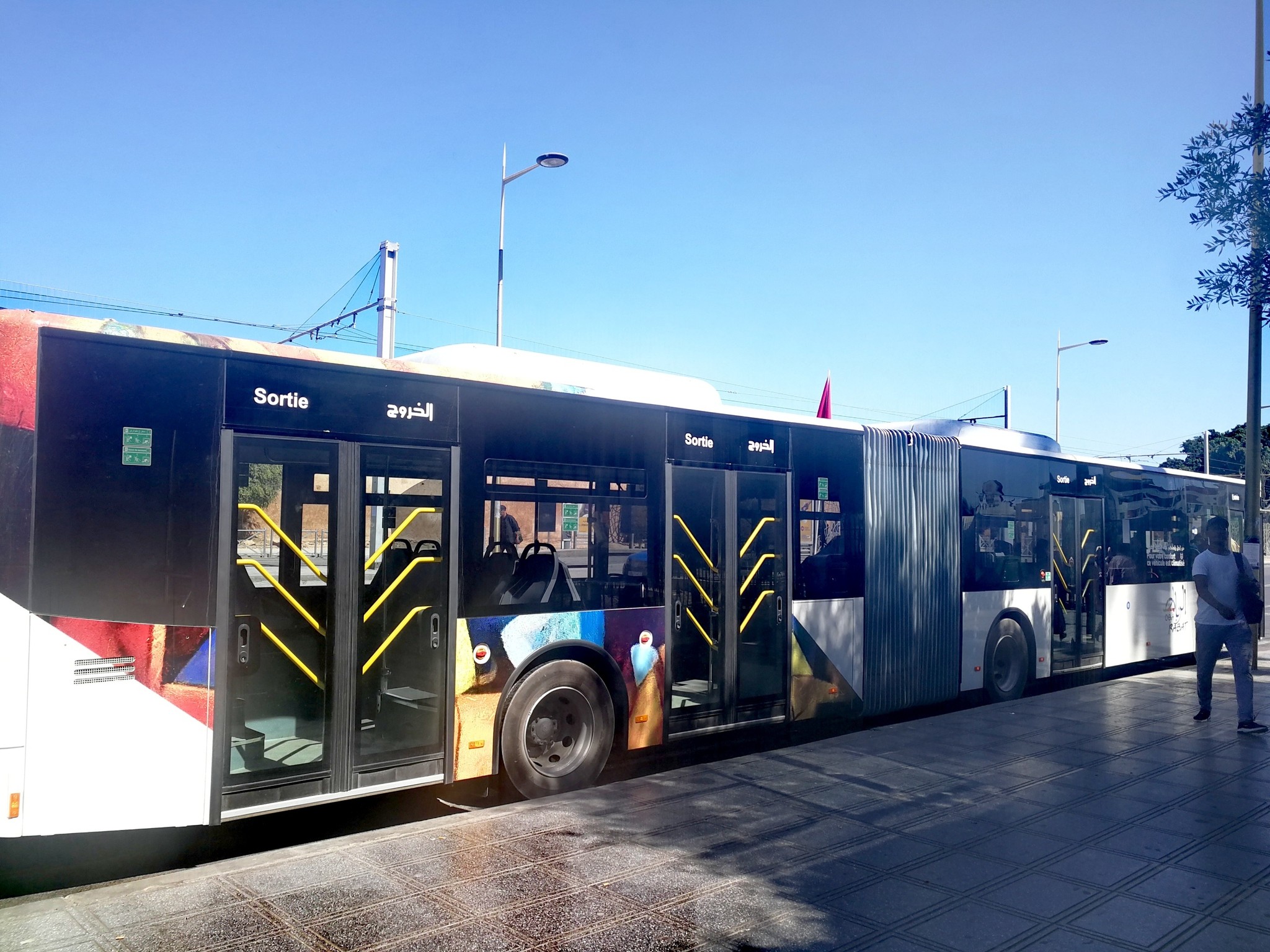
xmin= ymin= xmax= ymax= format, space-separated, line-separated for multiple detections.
xmin=1193 ymin=708 xmax=1211 ymax=722
xmin=1237 ymin=720 xmax=1268 ymax=734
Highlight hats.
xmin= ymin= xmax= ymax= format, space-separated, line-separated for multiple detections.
xmin=1206 ymin=516 xmax=1230 ymax=530
xmin=1096 ymin=546 xmax=1101 ymax=552
xmin=501 ymin=505 xmax=506 ymax=510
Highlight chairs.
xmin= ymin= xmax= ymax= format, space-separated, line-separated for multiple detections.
xmin=362 ymin=538 xmax=412 ymax=614
xmin=413 ymin=539 xmax=441 ymax=596
xmin=465 ymin=539 xmax=517 ymax=606
xmin=1139 ymin=571 xmax=1160 ymax=578
xmin=534 ymin=545 xmax=581 ymax=601
xmin=501 ymin=542 xmax=559 ymax=606
xmin=993 ymin=556 xmax=1024 ymax=584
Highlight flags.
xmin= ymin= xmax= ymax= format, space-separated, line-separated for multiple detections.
xmin=817 ymin=368 xmax=831 ymax=419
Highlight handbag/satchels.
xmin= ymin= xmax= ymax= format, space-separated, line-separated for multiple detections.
xmin=515 ymin=534 xmax=523 ymax=544
xmin=1233 ymin=551 xmax=1265 ymax=624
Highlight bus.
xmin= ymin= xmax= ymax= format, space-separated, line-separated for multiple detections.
xmin=1 ymin=297 xmax=1247 ymax=873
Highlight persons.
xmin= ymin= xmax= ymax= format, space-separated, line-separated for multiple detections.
xmin=500 ymin=505 xmax=522 ymax=558
xmin=1053 ymin=601 xmax=1067 ymax=641
xmin=1094 ymin=542 xmax=1139 ymax=641
xmin=960 ymin=511 xmax=1239 ymax=640
xmin=1192 ymin=517 xmax=1268 ymax=733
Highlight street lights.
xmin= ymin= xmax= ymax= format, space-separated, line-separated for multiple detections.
xmin=1053 ymin=338 xmax=1110 ymax=445
xmin=497 ymin=151 xmax=574 ymax=348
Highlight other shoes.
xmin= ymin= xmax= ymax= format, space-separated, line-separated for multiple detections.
xmin=1059 ymin=631 xmax=1067 ymax=641
